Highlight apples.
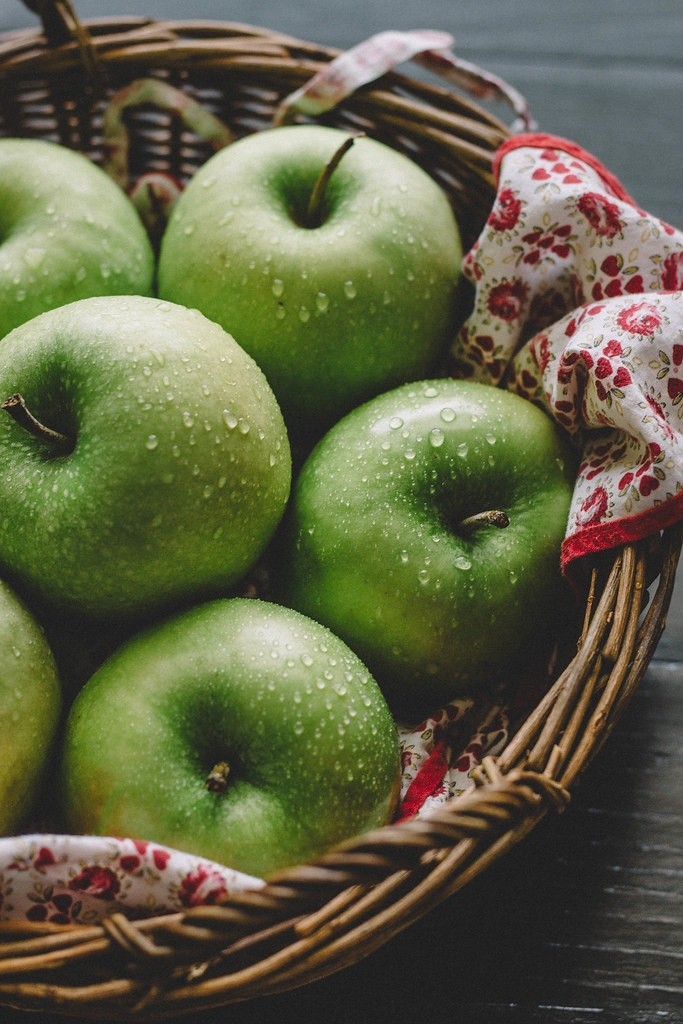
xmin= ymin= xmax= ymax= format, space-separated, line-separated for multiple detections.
xmin=1 ymin=576 xmax=63 ymax=839
xmin=156 ymin=123 xmax=464 ymax=429
xmin=1 ymin=296 xmax=295 ymax=625
xmin=47 ymin=598 xmax=406 ymax=880
xmin=275 ymin=376 xmax=579 ymax=690
xmin=0 ymin=138 xmax=157 ymax=339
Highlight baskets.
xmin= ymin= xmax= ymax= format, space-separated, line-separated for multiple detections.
xmin=0 ymin=0 xmax=683 ymax=1023
